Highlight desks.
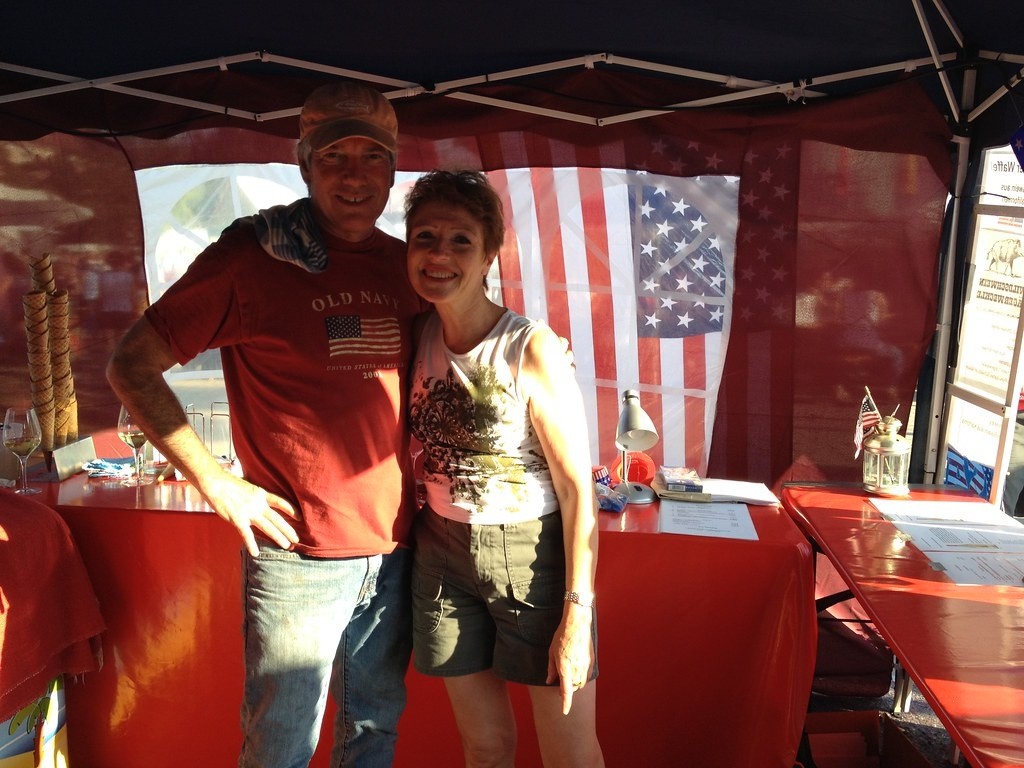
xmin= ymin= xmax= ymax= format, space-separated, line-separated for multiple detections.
xmin=781 ymin=480 xmax=1024 ymax=768
xmin=0 ymin=422 xmax=821 ymax=768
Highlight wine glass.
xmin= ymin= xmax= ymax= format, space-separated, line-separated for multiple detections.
xmin=117 ymin=402 xmax=154 ymax=487
xmin=2 ymin=407 xmax=43 ymax=496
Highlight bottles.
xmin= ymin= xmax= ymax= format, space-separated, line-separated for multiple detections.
xmin=864 ymin=415 xmax=910 ymax=496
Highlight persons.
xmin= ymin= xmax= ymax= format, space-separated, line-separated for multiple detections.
xmin=406 ymin=167 xmax=605 ymax=768
xmin=103 ymin=88 xmax=576 ymax=768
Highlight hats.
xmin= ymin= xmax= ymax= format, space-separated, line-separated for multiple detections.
xmin=299 ymin=82 xmax=398 ymax=155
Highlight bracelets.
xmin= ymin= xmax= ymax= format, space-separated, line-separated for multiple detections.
xmin=564 ymin=589 xmax=596 ymax=609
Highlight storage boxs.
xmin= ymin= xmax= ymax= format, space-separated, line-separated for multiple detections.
xmin=789 ymin=709 xmax=934 ymax=767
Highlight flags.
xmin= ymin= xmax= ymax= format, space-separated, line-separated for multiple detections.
xmin=475 ymin=124 xmax=799 ymax=478
xmin=853 ymin=395 xmax=881 ymax=460
